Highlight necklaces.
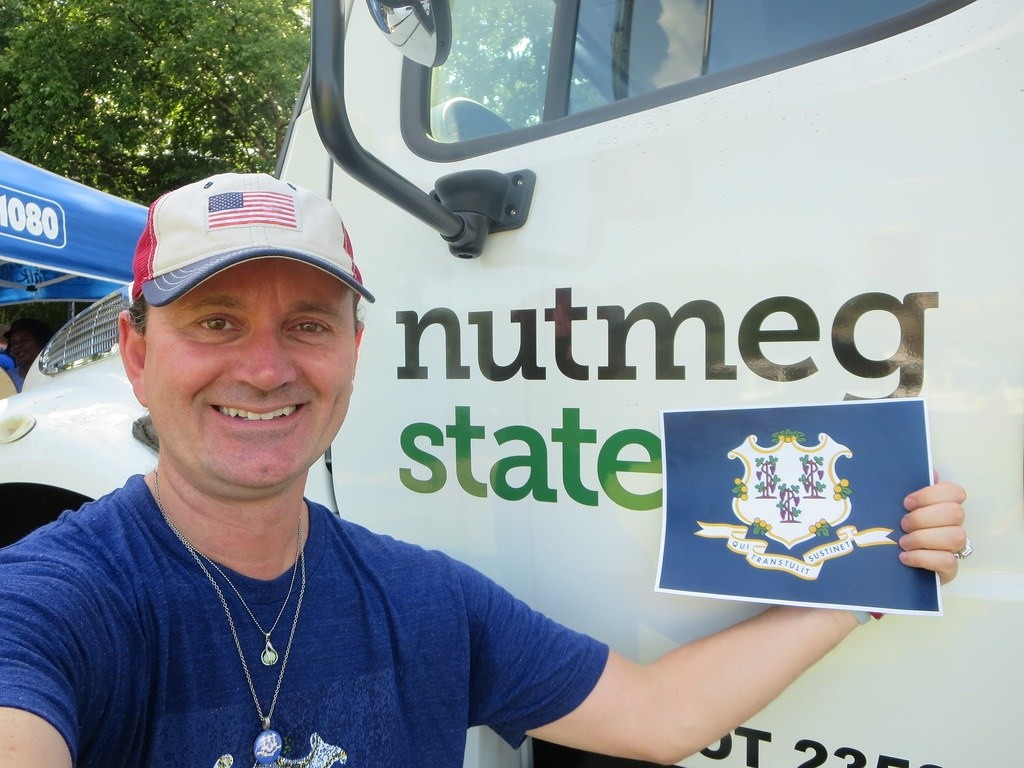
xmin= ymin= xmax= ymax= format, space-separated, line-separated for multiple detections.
xmin=150 ymin=467 xmax=305 ymax=766
xmin=156 ymin=467 xmax=300 ymax=666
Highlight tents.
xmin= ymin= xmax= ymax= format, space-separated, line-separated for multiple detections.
xmin=1 ymin=153 xmax=153 ymax=307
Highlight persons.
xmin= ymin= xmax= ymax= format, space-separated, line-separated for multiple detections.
xmin=0 ymin=317 xmax=59 ymax=401
xmin=0 ymin=171 xmax=968 ymax=768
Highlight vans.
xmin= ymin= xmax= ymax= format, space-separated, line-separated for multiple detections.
xmin=245 ymin=0 xmax=1024 ymax=768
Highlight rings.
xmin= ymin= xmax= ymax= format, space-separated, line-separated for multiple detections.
xmin=952 ymin=539 xmax=975 ymax=562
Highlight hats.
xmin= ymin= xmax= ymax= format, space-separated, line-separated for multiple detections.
xmin=132 ymin=172 xmax=375 ymax=307
xmin=3 ymin=318 xmax=50 ymax=341
